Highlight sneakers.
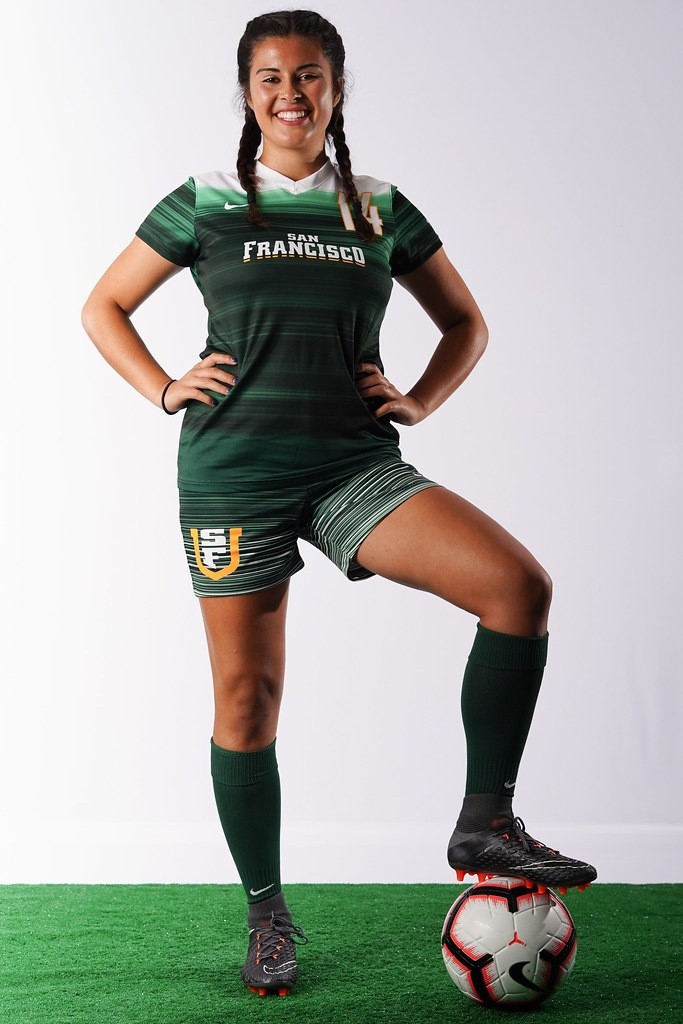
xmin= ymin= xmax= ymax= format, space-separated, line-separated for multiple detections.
xmin=245 ymin=890 xmax=306 ymax=995
xmin=447 ymin=794 xmax=596 ymax=895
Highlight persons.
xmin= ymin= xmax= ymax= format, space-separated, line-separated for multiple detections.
xmin=82 ymin=10 xmax=597 ymax=996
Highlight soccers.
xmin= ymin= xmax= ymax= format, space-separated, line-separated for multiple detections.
xmin=438 ymin=876 xmax=580 ymax=1012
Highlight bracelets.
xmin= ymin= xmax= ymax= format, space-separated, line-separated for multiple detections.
xmin=161 ymin=379 xmax=179 ymax=415
